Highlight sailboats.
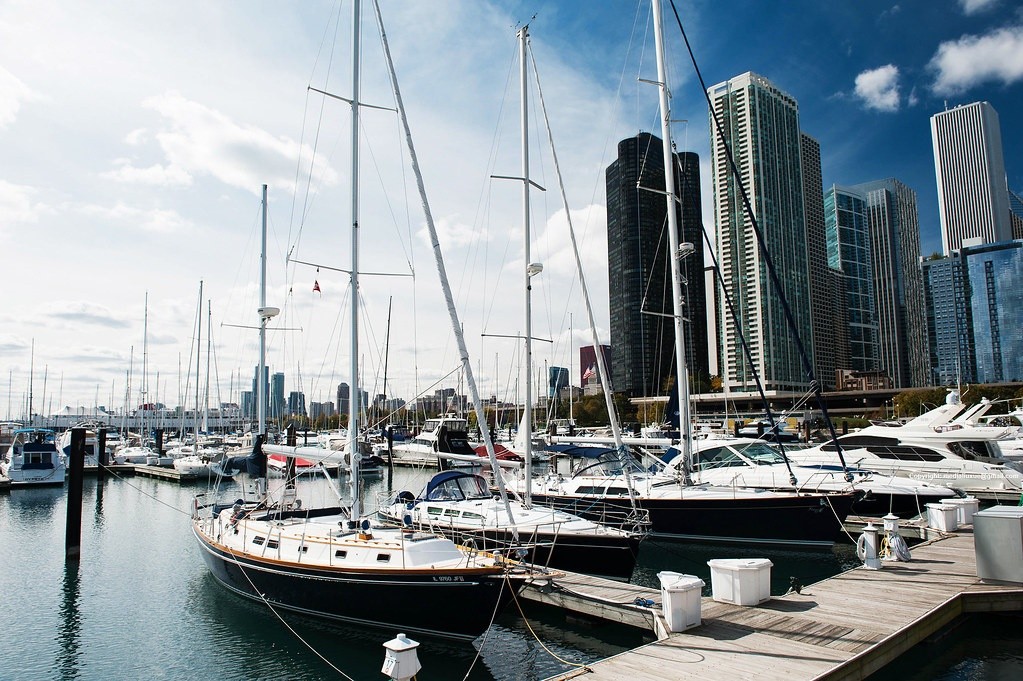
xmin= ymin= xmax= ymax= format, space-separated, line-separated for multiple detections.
xmin=1 ymin=2 xmax=1020 ymax=642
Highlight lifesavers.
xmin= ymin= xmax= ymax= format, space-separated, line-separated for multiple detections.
xmin=857 ymin=533 xmax=866 ymax=560
